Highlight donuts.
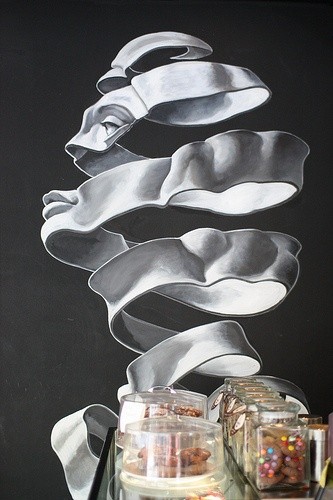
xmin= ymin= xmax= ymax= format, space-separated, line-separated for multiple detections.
xmin=136 ymin=445 xmax=212 ymax=478
xmin=256 ymin=432 xmax=307 ymax=490
